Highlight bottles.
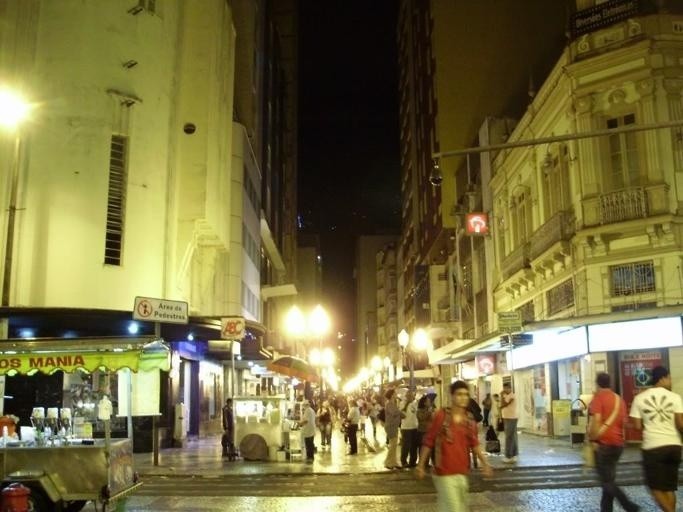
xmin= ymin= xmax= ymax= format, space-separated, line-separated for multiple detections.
xmin=3 ymin=426 xmax=8 ymax=445
xmin=32 ymin=407 xmax=72 ymax=438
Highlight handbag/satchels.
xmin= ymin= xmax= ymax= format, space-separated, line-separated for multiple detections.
xmin=584 ymin=444 xmax=596 ymax=467
xmin=487 ymin=439 xmax=500 ymax=452
xmin=376 ymin=408 xmax=384 ymax=423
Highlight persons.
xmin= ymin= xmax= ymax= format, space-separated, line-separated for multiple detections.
xmin=628 ymin=367 xmax=682 ymax=512
xmin=296 ymin=380 xmax=519 ymax=512
xmin=223 ymin=399 xmax=238 ymax=455
xmin=585 ymin=374 xmax=641 ymax=511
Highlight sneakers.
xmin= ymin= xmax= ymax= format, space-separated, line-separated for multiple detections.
xmin=504 ymin=456 xmax=517 ymax=463
xmin=384 ymin=463 xmax=417 ymax=470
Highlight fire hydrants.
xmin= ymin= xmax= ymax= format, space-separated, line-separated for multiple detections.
xmin=1 ymin=482 xmax=32 ymax=511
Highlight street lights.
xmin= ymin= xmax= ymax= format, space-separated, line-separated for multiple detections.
xmin=283 ymin=303 xmax=340 ymax=411
xmin=398 ymin=327 xmax=428 ymax=401
xmin=372 ymin=355 xmax=391 ymax=393
